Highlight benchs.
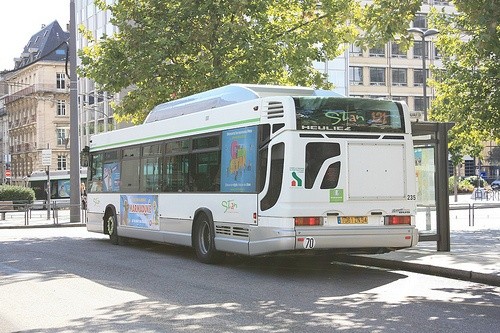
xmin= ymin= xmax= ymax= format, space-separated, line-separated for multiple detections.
xmin=0 ymin=200 xmax=19 ymax=221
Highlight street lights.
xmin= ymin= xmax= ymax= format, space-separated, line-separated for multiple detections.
xmin=405 ymin=27 xmax=439 ymax=120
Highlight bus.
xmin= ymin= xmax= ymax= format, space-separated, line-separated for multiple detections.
xmin=26 ymin=167 xmax=88 ymax=210
xmin=84 ymin=83 xmax=419 ymax=265
